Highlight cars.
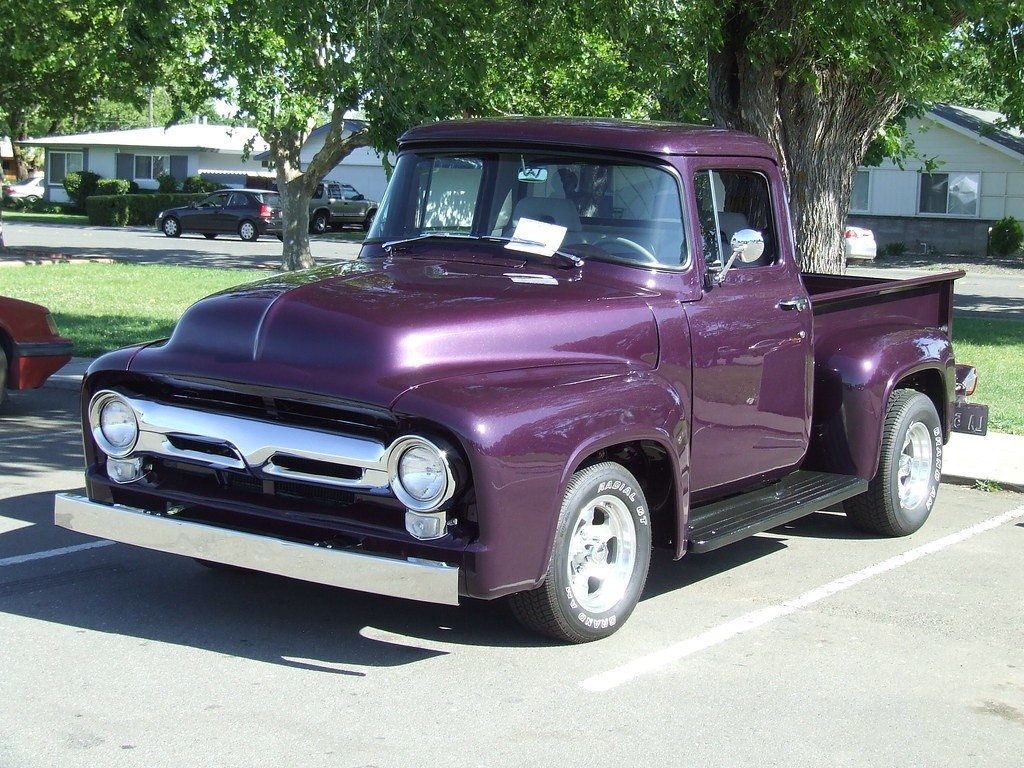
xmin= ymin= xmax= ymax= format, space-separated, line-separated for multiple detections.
xmin=155 ymin=188 xmax=283 ymax=242
xmin=844 ymin=225 xmax=878 ymax=266
xmin=0 ymin=297 xmax=75 ymax=408
xmin=4 ymin=176 xmax=80 ymax=206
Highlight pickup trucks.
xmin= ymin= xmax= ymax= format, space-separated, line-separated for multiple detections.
xmin=54 ymin=115 xmax=991 ymax=646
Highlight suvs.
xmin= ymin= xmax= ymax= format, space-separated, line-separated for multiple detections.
xmin=308 ymin=178 xmax=380 ymax=235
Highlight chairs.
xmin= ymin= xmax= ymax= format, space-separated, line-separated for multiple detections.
xmin=234 ymin=197 xmax=246 ymax=205
xmin=718 ymin=211 xmax=754 ymax=268
xmin=502 ymin=196 xmax=582 ymax=246
